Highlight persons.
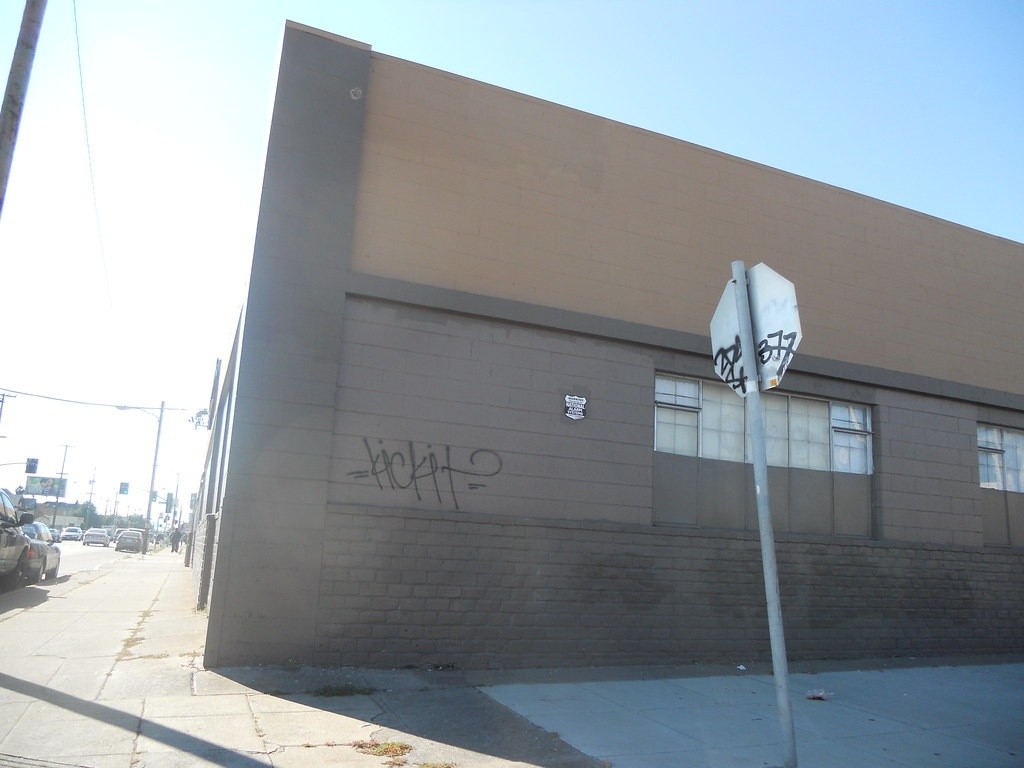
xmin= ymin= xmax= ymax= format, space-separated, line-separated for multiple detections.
xmin=29 ymin=478 xmax=48 ymax=495
xmin=42 ymin=478 xmax=57 ymax=496
xmin=169 ymin=528 xmax=188 ymax=553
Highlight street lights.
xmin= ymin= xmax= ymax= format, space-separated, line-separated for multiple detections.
xmin=117 ymin=401 xmax=166 ymax=556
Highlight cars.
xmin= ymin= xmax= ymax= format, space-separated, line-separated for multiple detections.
xmin=61 ymin=527 xmax=83 ymax=541
xmin=83 ymin=528 xmax=109 ymax=547
xmin=113 ymin=529 xmax=127 ymax=543
xmin=18 ymin=519 xmax=62 ymax=584
xmin=49 ymin=529 xmax=61 ymax=543
xmin=115 ymin=531 xmax=143 ymax=553
xmin=0 ymin=489 xmax=34 ymax=592
